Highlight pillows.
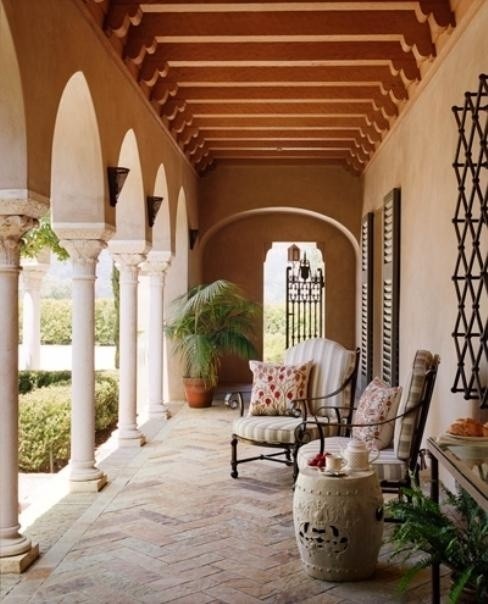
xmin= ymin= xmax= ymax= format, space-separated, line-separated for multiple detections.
xmin=248 ymin=358 xmax=316 ymax=418
xmin=350 ymin=374 xmax=403 ymax=450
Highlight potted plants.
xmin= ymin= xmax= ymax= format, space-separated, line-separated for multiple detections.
xmin=375 ymin=475 xmax=488 ymax=604
xmin=163 ymin=278 xmax=262 ymax=408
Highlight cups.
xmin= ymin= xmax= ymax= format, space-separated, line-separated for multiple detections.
xmin=326 ymin=457 xmax=345 ymax=472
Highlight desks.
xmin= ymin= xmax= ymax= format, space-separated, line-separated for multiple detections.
xmin=291 ymin=464 xmax=383 ymax=583
xmin=426 ymin=421 xmax=487 ymax=603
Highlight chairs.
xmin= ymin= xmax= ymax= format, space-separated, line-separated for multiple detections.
xmin=292 ymin=348 xmax=441 ymax=508
xmin=229 ymin=338 xmax=365 ymax=491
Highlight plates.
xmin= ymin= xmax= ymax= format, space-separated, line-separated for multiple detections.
xmin=444 ymin=432 xmax=488 ymax=442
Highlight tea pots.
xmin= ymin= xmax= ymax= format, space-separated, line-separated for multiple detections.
xmin=338 ymin=438 xmax=381 ymax=472
